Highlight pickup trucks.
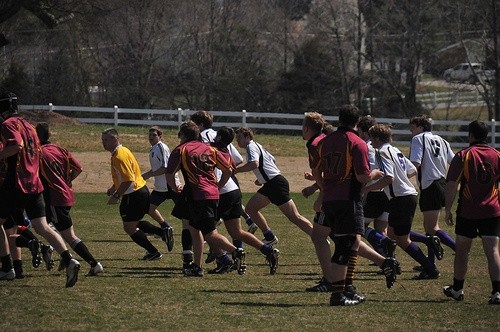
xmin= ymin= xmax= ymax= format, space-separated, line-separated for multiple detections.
xmin=444 ymin=62 xmax=496 ymax=85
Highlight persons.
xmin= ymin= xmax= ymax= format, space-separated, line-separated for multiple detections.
xmin=317 ymin=103 xmax=385 ymax=307
xmin=443 ymin=120 xmax=500 ymax=304
xmin=235 ymin=127 xmax=331 ymax=247
xmin=141 ymin=111 xmax=279 ymax=277
xmin=0 ymin=91 xmax=104 ymax=288
xmin=101 ymin=127 xmax=174 ymax=261
xmin=302 ymin=111 xmax=457 ymax=290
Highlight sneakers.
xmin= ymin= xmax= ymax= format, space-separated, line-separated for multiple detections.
xmin=329 ymin=288 xmax=366 ymax=305
xmin=161 ymin=227 xmax=176 ymax=251
xmin=305 ymin=280 xmax=331 ymax=292
xmin=416 ymin=270 xmax=441 ymax=279
xmin=0 ymin=239 xmax=104 ymax=288
xmin=141 ymin=252 xmax=164 ymax=261
xmin=442 ymin=286 xmax=465 ymax=300
xmin=428 ymin=236 xmax=444 ymax=261
xmin=386 ymin=239 xmax=397 ymax=258
xmin=178 ymin=224 xmax=281 ymax=277
xmin=488 ymin=292 xmax=500 ymax=304
xmin=382 ymin=258 xmax=398 ymax=288
